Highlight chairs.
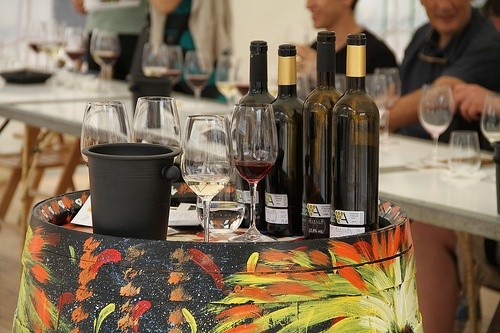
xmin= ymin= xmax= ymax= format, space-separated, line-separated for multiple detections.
xmin=0 ymin=126 xmax=91 ymax=232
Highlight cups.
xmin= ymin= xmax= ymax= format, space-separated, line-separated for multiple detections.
xmin=196 ymin=160 xmax=245 ymax=235
xmin=80 ymin=101 xmax=132 ymax=168
xmin=235 ymin=52 xmax=249 ymax=96
xmin=448 ymin=130 xmax=481 ymax=173
xmin=298 ymin=71 xmax=387 ymax=120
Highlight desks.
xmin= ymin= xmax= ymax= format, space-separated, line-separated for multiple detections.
xmin=377 ymin=153 xmax=500 ymax=333
xmin=10 ymin=95 xmax=481 ymax=263
xmin=0 ymin=71 xmax=137 ymax=118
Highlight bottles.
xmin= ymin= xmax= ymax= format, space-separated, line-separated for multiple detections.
xmin=257 ymin=43 xmax=304 ymax=238
xmin=329 ymin=33 xmax=380 ymax=237
xmin=231 ymin=41 xmax=276 ymax=229
xmin=300 ymin=30 xmax=343 ymax=238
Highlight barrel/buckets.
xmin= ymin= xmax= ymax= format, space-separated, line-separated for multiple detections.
xmin=82 ymin=142 xmax=181 ymax=241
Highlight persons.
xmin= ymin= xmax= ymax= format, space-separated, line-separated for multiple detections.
xmin=72 ymin=0 xmax=150 ymax=78
xmin=481 ymin=0 xmax=500 ymax=28
xmin=288 ymin=0 xmax=399 ymax=87
xmin=135 ymin=0 xmax=237 ymax=102
xmin=363 ymin=0 xmax=500 ymax=333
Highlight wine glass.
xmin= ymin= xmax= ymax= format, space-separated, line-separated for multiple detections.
xmin=480 ymin=94 xmax=500 ymax=184
xmin=418 ymin=84 xmax=453 ymax=168
xmin=373 ymin=68 xmax=401 ymax=146
xmin=180 ymin=114 xmax=234 ymax=243
xmin=143 ymin=41 xmax=238 ymax=115
xmin=226 ymin=104 xmax=277 ymax=242
xmin=132 ymin=96 xmax=183 ymax=236
xmin=26 ymin=19 xmax=119 ymax=94
